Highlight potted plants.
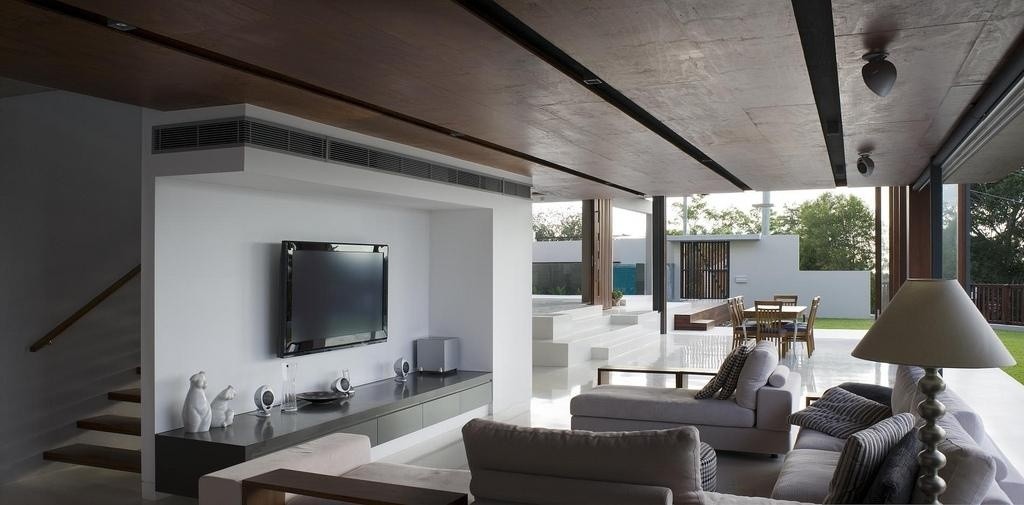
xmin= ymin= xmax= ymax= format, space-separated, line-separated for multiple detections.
xmin=612 ymin=288 xmax=623 ymax=306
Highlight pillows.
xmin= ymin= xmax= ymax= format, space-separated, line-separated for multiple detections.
xmin=821 ymin=412 xmax=915 ymax=504
xmin=717 ymin=339 xmax=756 ymax=402
xmin=865 ymin=427 xmax=924 ymax=504
xmin=786 ymin=385 xmax=891 ymax=440
xmin=699 ymin=444 xmax=719 ymax=493
xmin=695 ymin=345 xmax=737 ymax=398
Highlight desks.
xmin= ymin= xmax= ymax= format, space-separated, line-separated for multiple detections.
xmin=742 ymin=305 xmax=807 ymax=360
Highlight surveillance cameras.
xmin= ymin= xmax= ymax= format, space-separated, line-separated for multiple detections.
xmin=856 ymin=151 xmax=873 ymax=176
xmin=861 ymin=53 xmax=897 ymax=97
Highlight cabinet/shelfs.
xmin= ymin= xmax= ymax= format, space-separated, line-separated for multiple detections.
xmin=156 ymin=371 xmax=491 ymax=496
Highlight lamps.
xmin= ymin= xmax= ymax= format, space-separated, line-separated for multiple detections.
xmin=852 ymin=279 xmax=1017 ymax=505
xmin=860 ymin=50 xmax=897 ymax=100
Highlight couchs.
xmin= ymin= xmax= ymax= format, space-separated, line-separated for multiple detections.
xmin=570 ymin=340 xmax=800 ymax=462
xmin=198 ymin=419 xmax=825 ymax=505
xmin=772 ymin=366 xmax=1012 ymax=505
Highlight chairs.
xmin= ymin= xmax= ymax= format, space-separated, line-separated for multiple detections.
xmin=755 ymin=300 xmax=787 ymax=361
xmin=779 ymin=296 xmax=821 ymax=357
xmin=774 ymin=295 xmax=798 ymax=306
xmin=727 ymin=295 xmax=767 ymax=350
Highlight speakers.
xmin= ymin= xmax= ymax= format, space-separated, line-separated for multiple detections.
xmin=331 ymin=377 xmax=352 ymax=393
xmin=254 ymin=385 xmax=276 ymax=417
xmin=394 ymin=357 xmax=412 ymax=382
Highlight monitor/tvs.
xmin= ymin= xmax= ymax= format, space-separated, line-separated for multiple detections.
xmin=277 ymin=240 xmax=389 ymax=359
